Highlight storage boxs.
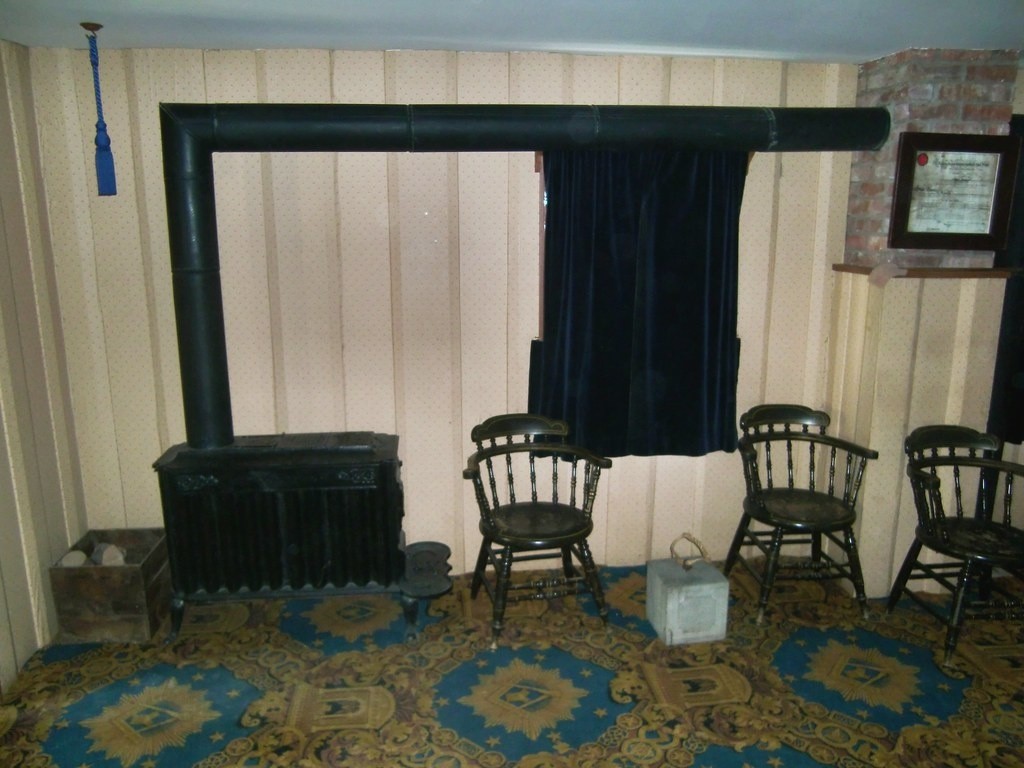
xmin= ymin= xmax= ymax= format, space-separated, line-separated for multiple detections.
xmin=50 ymin=528 xmax=174 ymax=642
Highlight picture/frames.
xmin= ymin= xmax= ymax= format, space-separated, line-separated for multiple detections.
xmin=887 ymin=131 xmax=1022 ymax=251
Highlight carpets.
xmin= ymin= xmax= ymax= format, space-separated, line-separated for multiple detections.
xmin=0 ymin=555 xmax=1024 ymax=768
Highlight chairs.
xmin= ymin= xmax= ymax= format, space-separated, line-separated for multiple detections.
xmin=723 ymin=404 xmax=878 ymax=625
xmin=463 ymin=414 xmax=614 ymax=652
xmin=880 ymin=425 xmax=1024 ymax=666
